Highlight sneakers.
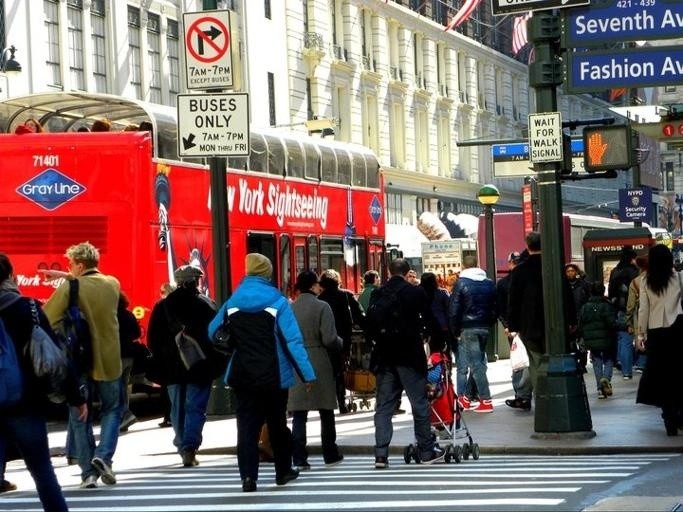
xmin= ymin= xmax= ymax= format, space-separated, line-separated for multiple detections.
xmin=598 ymin=389 xmax=604 ymax=398
xmin=180 ymin=449 xmax=198 ymax=466
xmin=457 ymin=395 xmax=470 ymax=409
xmin=473 ymin=396 xmax=493 ymax=412
xmin=600 ymin=375 xmax=611 ymax=395
xmin=325 ymin=454 xmax=342 ymax=466
xmin=295 ymin=462 xmax=309 ymax=470
xmin=91 ymin=456 xmax=115 ymax=484
xmin=120 ymin=410 xmax=136 ymax=429
xmin=79 ymin=474 xmax=97 ymax=488
xmin=506 ymin=399 xmax=530 ymax=409
xmin=375 ymin=457 xmax=386 ymax=467
xmin=420 ymin=429 xmax=466 ymax=464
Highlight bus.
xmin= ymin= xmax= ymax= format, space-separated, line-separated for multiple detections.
xmin=477 ymin=210 xmax=671 ymax=286
xmin=0 ymin=90 xmax=390 ymax=346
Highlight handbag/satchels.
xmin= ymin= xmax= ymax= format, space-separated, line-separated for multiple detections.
xmin=211 ymin=323 xmax=233 ymax=355
xmin=175 ymin=326 xmax=206 ymax=370
xmin=29 ymin=325 xmax=67 ymax=382
xmin=63 ymin=306 xmax=94 ymax=364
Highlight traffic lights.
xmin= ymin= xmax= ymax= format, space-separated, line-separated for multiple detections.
xmin=580 ymin=124 xmax=632 ymax=173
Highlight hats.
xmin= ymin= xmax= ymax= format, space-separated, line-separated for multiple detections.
xmin=426 ymin=353 xmax=448 ymax=369
xmin=173 ymin=265 xmax=203 ymax=282
xmin=246 ymin=253 xmax=272 ymax=276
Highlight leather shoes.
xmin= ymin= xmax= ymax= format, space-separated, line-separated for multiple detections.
xmin=276 ymin=466 xmax=299 ymax=485
xmin=243 ymin=477 xmax=255 ymax=491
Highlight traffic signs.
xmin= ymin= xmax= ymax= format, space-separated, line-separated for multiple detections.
xmin=490 ymin=1 xmax=592 ymax=16
xmin=565 ymin=45 xmax=682 ymax=94
xmin=563 ymin=0 xmax=682 ymax=46
xmin=177 ymin=93 xmax=251 ymax=157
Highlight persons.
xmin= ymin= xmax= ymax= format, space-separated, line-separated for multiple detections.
xmin=0 ymin=463 xmax=18 ymax=492
xmin=44 ymin=240 xmax=123 ymax=489
xmin=117 ymin=293 xmax=141 ymax=432
xmin=0 ymin=254 xmax=89 ymax=512
xmin=505 ymin=231 xmax=683 ymax=438
xmin=66 ymin=421 xmax=78 ymax=465
xmin=148 ymin=252 xmax=498 ymax=492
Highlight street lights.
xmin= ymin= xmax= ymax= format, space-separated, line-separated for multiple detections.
xmin=0 ymin=44 xmax=22 ymax=77
xmin=472 ymin=182 xmax=501 ymax=284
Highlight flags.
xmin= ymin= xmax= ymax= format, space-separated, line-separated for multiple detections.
xmin=443 ymin=0 xmax=482 ymax=33
xmin=512 ymin=14 xmax=528 ymax=54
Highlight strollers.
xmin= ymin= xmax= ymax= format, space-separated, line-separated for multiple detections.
xmin=401 ymin=340 xmax=480 ymax=463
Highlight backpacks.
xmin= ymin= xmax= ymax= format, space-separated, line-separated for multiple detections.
xmin=0 ymin=292 xmax=20 ymax=402
